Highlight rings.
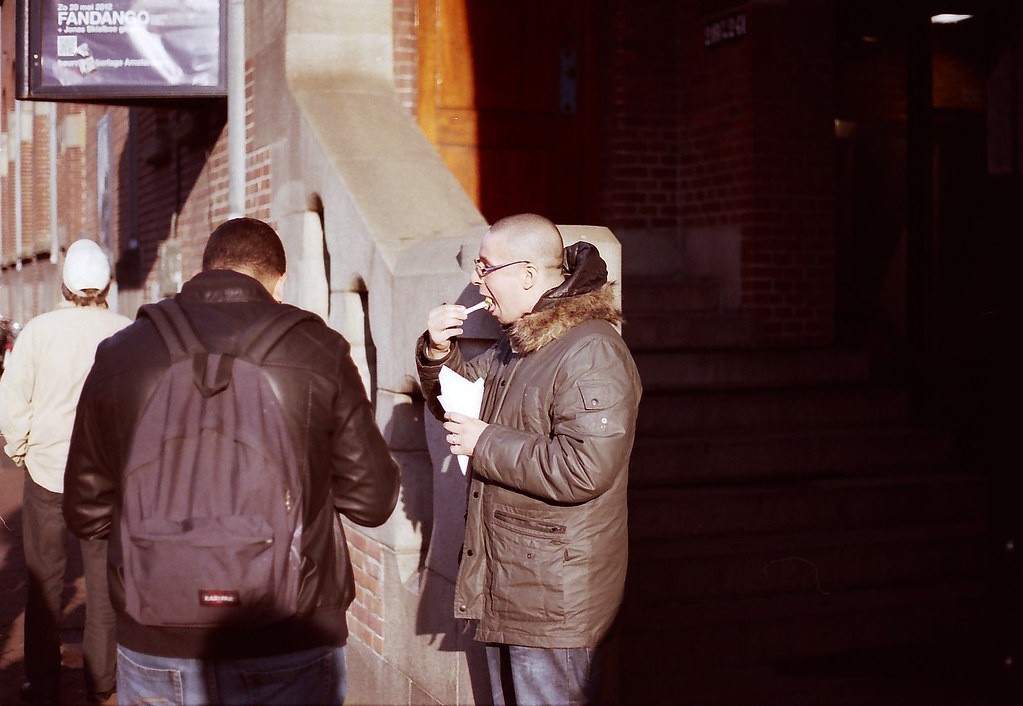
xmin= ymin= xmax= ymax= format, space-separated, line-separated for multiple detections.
xmin=452 ymin=434 xmax=456 ymax=445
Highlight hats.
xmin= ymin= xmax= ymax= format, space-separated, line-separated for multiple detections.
xmin=63 ymin=238 xmax=111 ymax=296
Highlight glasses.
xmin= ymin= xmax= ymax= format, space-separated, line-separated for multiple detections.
xmin=474 ymin=256 xmax=531 ymax=280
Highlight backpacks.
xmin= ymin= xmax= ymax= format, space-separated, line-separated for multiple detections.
xmin=119 ymin=299 xmax=302 ymax=626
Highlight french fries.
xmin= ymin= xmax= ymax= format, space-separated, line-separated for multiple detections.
xmin=466 ymin=301 xmax=487 ymax=314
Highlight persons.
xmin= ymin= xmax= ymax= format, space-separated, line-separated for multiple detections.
xmin=414 ymin=215 xmax=641 ymax=706
xmin=2 ymin=238 xmax=137 ymax=706
xmin=61 ymin=219 xmax=401 ymax=706
